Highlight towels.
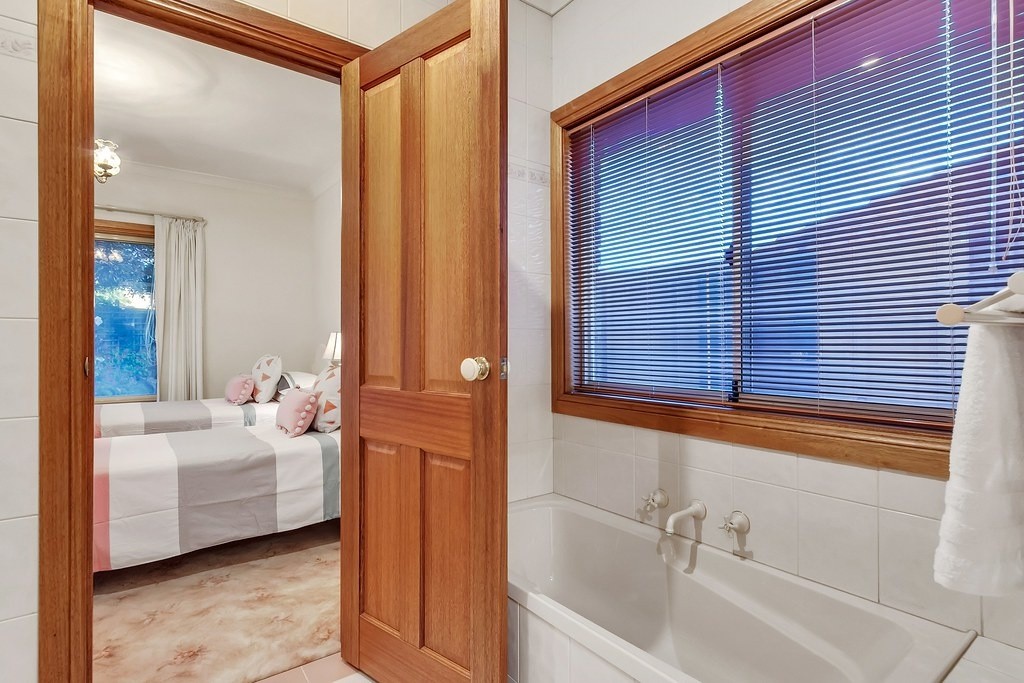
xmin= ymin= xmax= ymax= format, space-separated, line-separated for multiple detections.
xmin=934 ymin=271 xmax=1024 ymax=597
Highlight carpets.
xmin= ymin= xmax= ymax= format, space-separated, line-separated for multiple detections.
xmin=93 ymin=522 xmax=341 ymax=682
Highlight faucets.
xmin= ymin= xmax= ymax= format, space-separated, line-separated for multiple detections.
xmin=665 ymin=499 xmax=707 ymax=536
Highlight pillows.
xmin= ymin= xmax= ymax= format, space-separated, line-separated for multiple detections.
xmin=275 ymin=383 xmax=318 ymax=438
xmin=251 ymin=353 xmax=282 ymax=404
xmin=272 ymin=371 xmax=318 ymax=403
xmin=310 ymin=362 xmax=341 ymax=434
xmin=224 ymin=373 xmax=254 ymax=406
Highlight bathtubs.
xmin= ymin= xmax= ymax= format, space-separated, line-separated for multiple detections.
xmin=470 ymin=490 xmax=980 ymax=683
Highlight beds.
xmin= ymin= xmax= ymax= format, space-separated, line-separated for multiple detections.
xmin=93 ymin=396 xmax=281 ymax=438
xmin=94 ymin=424 xmax=341 ymax=573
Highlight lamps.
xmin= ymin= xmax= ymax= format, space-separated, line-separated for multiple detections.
xmin=322 ymin=331 xmax=342 ymax=365
xmin=94 ymin=138 xmax=121 ymax=183
